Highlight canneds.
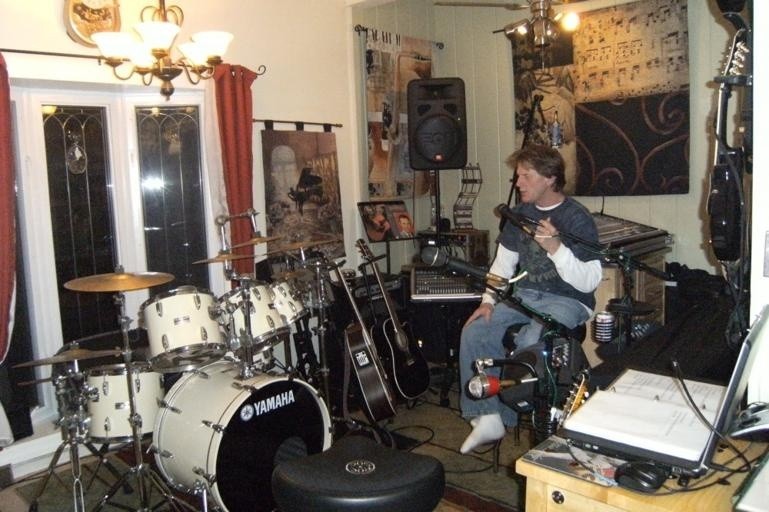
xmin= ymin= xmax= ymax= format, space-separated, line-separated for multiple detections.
xmin=595 ymin=311 xmax=615 ymax=342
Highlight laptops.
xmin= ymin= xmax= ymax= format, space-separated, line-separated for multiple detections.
xmin=555 ymin=302 xmax=769 ymax=479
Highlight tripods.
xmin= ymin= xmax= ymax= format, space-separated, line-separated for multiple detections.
xmin=89 ymin=294 xmax=200 ymax=512
xmin=28 ymin=360 xmax=134 ymax=512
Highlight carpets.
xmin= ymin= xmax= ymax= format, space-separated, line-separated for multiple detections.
xmin=13 ymin=453 xmax=171 ymax=510
xmin=115 ymin=441 xmax=515 ymax=511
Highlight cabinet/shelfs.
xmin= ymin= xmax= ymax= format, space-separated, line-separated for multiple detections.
xmin=515 ymin=433 xmax=768 ymax=511
xmin=580 ymin=246 xmax=674 ymax=368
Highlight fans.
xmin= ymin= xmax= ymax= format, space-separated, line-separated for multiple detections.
xmin=430 ymin=0 xmax=593 ymax=74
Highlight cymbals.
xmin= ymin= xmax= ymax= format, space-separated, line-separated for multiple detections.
xmin=227 ymin=237 xmax=277 ymax=250
xmin=64 ymin=272 xmax=175 ymax=293
xmin=9 ymin=350 xmax=128 ymax=368
xmin=266 ymin=241 xmax=331 ymax=255
xmin=192 ymin=254 xmax=254 ymax=266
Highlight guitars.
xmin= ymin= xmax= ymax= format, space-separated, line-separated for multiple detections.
xmin=356 ymin=239 xmax=430 ymax=399
xmin=706 ymin=13 xmax=750 ymax=263
xmin=329 ymin=262 xmax=398 ymax=423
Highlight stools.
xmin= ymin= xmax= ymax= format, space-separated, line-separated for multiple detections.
xmin=496 ymin=320 xmax=588 ymax=475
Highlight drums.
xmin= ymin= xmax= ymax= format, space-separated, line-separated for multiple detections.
xmin=152 ymin=361 xmax=332 ymax=512
xmin=268 ymin=281 xmax=306 ymax=326
xmin=85 ymin=360 xmax=163 ymax=444
xmin=217 ymin=280 xmax=290 ymax=359
xmin=139 ymin=286 xmax=225 ymax=373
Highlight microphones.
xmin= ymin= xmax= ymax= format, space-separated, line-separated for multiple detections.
xmin=467 ymin=374 xmax=538 ymax=399
xmin=497 ymin=202 xmax=536 ymax=238
xmin=420 ymin=246 xmax=507 ymax=285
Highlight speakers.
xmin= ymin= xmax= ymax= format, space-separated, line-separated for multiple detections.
xmin=406 ymin=77 xmax=467 ymax=170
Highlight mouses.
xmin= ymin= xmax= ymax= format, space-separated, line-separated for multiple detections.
xmin=614 ymin=463 xmax=665 ymax=493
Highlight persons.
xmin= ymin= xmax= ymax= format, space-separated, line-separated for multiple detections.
xmin=363 ymin=204 xmax=414 ymax=242
xmin=459 ymin=146 xmax=602 ymax=455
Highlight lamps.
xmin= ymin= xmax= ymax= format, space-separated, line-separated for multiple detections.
xmin=88 ymin=1 xmax=235 ymax=102
xmin=502 ymin=10 xmax=581 ymax=47
